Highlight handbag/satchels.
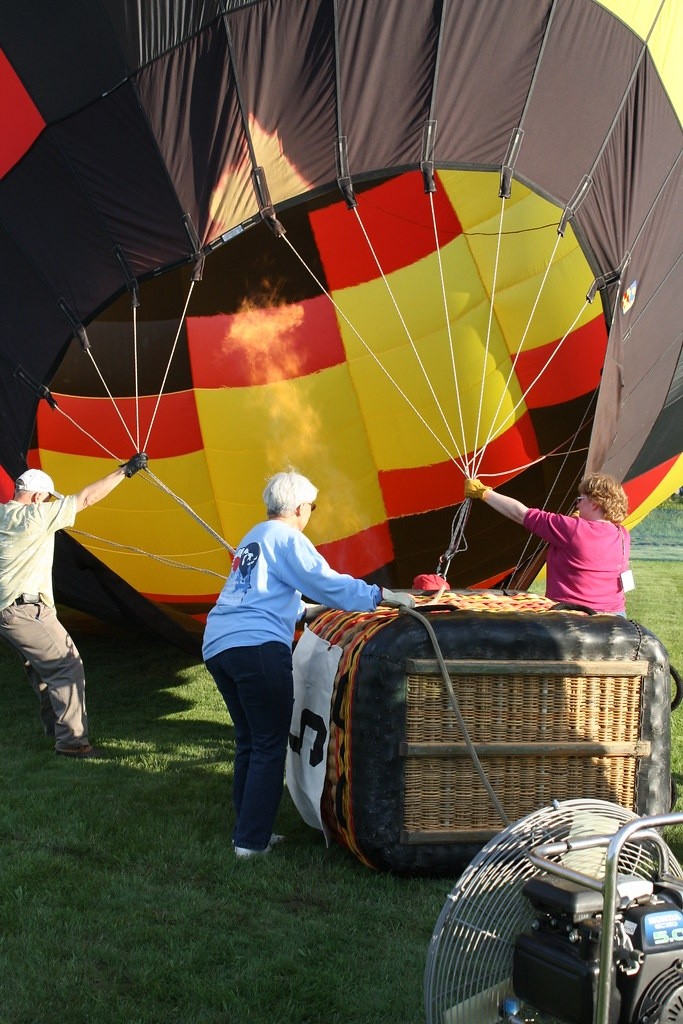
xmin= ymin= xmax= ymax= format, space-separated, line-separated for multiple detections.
xmin=620 ymin=570 xmax=635 ymax=593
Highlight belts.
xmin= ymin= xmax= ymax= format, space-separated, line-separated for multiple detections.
xmin=10 ymin=597 xmax=23 ymax=606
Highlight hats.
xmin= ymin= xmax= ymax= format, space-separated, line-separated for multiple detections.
xmin=16 ymin=469 xmax=64 ymax=500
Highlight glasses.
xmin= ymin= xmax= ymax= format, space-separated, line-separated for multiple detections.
xmin=308 ymin=503 xmax=316 ymax=511
xmin=576 ymin=496 xmax=590 ymax=503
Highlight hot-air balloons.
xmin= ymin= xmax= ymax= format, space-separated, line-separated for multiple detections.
xmin=2 ymin=3 xmax=683 ymax=878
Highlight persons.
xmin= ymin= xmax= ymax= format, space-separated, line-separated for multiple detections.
xmin=201 ymin=472 xmax=416 ymax=858
xmin=0 ymin=451 xmax=149 ymax=759
xmin=464 ymin=471 xmax=632 ymax=619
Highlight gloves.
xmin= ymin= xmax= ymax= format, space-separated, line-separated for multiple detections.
xmin=119 ymin=452 xmax=148 ymax=478
xmin=464 ymin=479 xmax=493 ymax=500
xmin=305 ymin=603 xmax=327 ymax=619
xmin=378 ymin=587 xmax=416 ymax=614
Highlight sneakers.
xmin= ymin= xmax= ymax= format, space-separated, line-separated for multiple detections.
xmin=235 ymin=845 xmax=272 ymax=859
xmin=55 ymin=745 xmax=103 ymax=758
xmin=232 ymin=833 xmax=287 ymax=846
